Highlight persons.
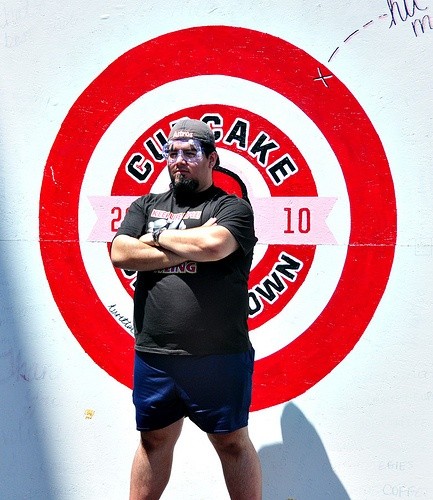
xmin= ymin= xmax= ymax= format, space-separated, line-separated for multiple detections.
xmin=111 ymin=122 xmax=264 ymax=500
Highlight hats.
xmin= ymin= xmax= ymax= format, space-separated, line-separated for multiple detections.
xmin=168 ymin=119 xmax=220 ymax=165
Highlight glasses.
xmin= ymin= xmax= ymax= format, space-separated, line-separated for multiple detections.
xmin=163 ymin=141 xmax=205 ymax=162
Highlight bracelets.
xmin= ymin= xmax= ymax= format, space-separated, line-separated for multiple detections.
xmin=152 ymin=228 xmax=162 ymax=244
